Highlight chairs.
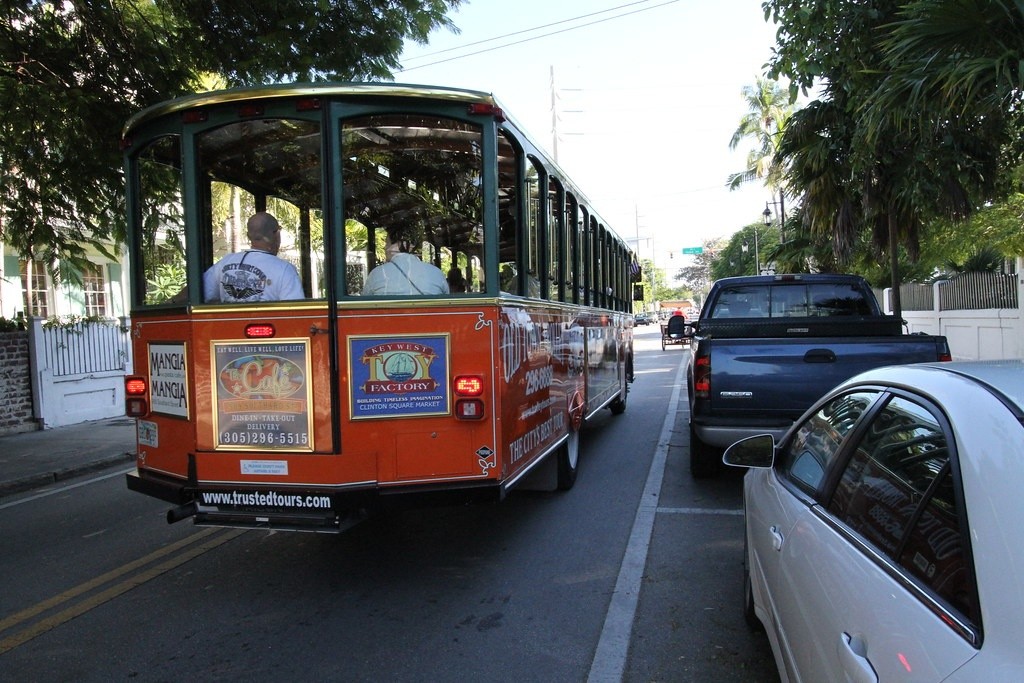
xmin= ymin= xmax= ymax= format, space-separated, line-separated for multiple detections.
xmin=814 ymin=294 xmax=839 ymax=315
xmin=728 ymin=301 xmax=752 ymax=316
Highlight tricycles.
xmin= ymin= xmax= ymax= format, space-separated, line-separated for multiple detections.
xmin=660 ymin=320 xmax=693 ymax=352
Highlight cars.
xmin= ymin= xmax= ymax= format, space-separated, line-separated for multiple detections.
xmin=721 ymin=358 xmax=1023 ymax=683
xmin=634 ymin=309 xmax=668 ymax=327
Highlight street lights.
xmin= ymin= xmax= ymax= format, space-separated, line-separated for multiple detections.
xmin=741 ymin=227 xmax=759 ymax=276
xmin=762 ymin=186 xmax=785 ymax=244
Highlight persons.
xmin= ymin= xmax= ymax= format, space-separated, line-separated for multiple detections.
xmin=359 ymin=218 xmax=449 ymax=296
xmin=670 ymin=307 xmax=688 ymax=319
xmin=444 ymin=258 xmax=541 ymax=298
xmin=169 ymin=212 xmax=306 ymax=302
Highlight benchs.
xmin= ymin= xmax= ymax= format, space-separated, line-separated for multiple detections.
xmin=659 ymin=324 xmax=693 ymax=338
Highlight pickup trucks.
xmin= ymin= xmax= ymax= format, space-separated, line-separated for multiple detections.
xmin=667 ymin=273 xmax=953 ymax=476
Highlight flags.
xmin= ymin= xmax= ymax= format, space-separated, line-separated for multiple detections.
xmin=630 ymin=254 xmax=640 ymax=276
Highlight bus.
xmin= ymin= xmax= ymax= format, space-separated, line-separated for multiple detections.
xmin=122 ymin=81 xmax=645 ymax=535
